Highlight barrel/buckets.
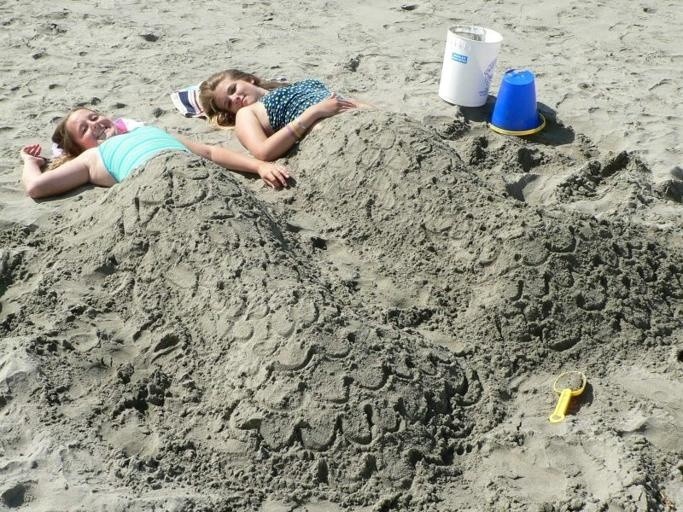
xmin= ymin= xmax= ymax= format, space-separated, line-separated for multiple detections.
xmin=486 ymin=70 xmax=547 ymax=137
xmin=439 ymin=25 xmax=503 ymax=107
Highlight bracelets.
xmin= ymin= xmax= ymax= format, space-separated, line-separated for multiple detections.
xmin=294 ymin=118 xmax=307 ymax=132
xmin=290 ymin=123 xmax=302 ymax=139
xmin=286 ymin=126 xmax=299 ymax=144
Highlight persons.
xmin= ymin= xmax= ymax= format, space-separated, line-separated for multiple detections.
xmin=20 ymin=106 xmax=289 ymax=200
xmin=198 ymin=69 xmax=373 ymax=162
